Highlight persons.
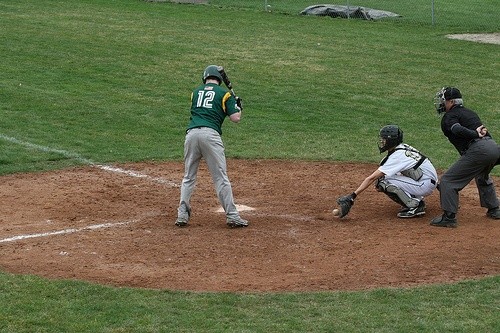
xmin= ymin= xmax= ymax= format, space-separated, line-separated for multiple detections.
xmin=175 ymin=64 xmax=250 ymax=228
xmin=337 ymin=125 xmax=438 ymax=218
xmin=430 ymin=86 xmax=500 ymax=227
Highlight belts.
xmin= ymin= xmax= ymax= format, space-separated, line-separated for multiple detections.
xmin=431 ymin=179 xmax=435 ymax=185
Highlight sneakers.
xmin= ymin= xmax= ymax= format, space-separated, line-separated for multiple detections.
xmin=430 ymin=212 xmax=457 ymax=228
xmin=174 ymin=216 xmax=188 ymax=225
xmin=486 ymin=207 xmax=500 ymax=218
xmin=397 ymin=204 xmax=427 ymax=217
xmin=226 ymin=215 xmax=248 ymax=226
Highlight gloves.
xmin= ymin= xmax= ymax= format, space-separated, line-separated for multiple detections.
xmin=234 ymin=96 xmax=243 ymax=111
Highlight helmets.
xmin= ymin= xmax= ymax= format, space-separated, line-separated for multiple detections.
xmin=378 ymin=125 xmax=404 ymax=152
xmin=203 ymin=65 xmax=223 ymax=84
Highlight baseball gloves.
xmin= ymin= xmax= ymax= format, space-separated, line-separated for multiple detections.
xmin=336 ymin=195 xmax=354 ymax=218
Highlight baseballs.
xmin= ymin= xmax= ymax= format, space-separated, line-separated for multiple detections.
xmin=333 ymin=209 xmax=339 ymax=216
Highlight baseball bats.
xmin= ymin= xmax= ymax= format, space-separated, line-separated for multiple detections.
xmin=217 ymin=66 xmax=238 ymax=100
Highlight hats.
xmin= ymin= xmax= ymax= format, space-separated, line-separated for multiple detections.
xmin=438 ymin=88 xmax=463 ymax=99
